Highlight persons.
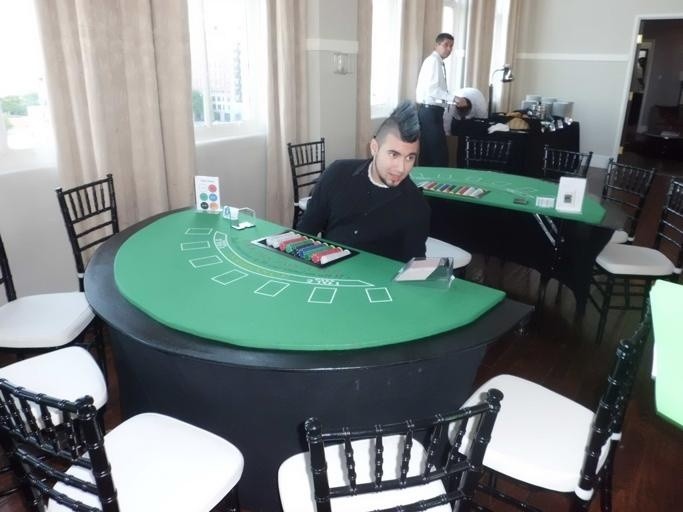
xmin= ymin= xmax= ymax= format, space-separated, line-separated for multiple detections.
xmin=414 ymin=32 xmax=468 ymax=166
xmin=294 ymin=99 xmax=430 ymax=264
xmin=446 ymin=87 xmax=489 ymax=170
xmin=627 ymin=56 xmax=645 ymax=128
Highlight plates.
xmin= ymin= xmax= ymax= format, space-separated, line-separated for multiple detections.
xmin=521 ymin=95 xmax=575 ymax=120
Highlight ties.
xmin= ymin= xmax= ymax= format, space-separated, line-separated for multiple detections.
xmin=442 ymin=62 xmax=447 ymax=91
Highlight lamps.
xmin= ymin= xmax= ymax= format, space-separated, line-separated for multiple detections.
xmin=488 ymin=64 xmax=516 ymax=113
